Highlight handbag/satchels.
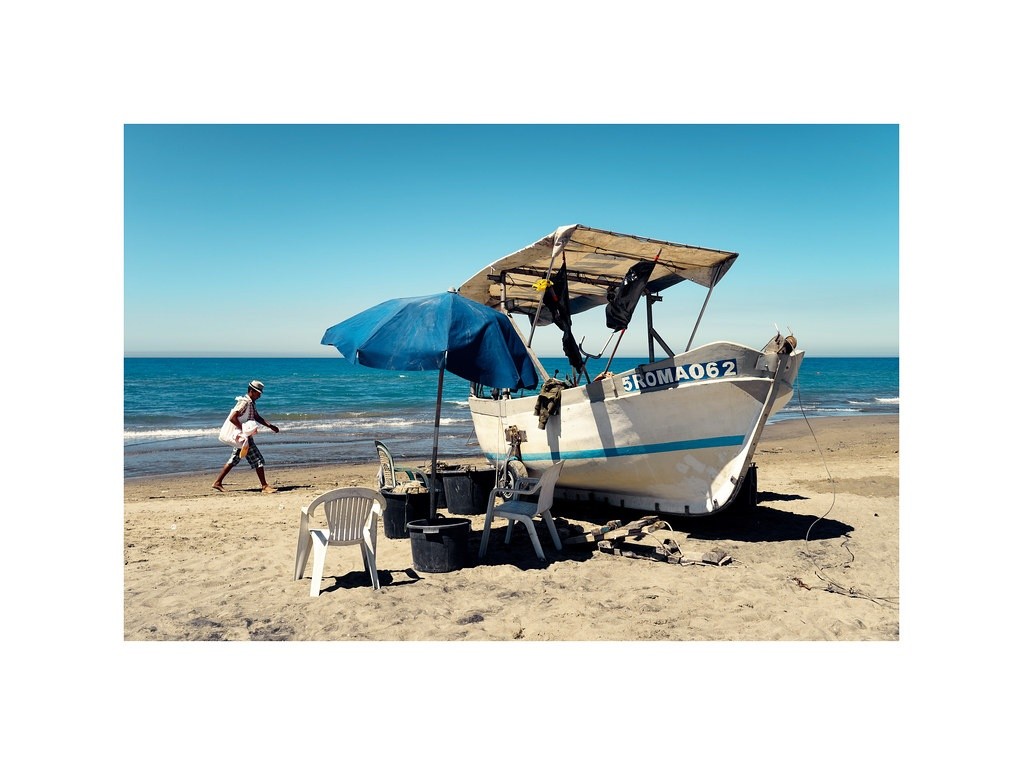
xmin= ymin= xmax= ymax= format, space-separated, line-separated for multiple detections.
xmin=218 ymin=396 xmax=251 ymax=449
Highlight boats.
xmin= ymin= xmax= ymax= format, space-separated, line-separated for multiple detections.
xmin=455 ymin=224 xmax=805 ymax=520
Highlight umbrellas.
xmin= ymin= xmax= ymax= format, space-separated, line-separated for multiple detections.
xmin=320 ymin=285 xmax=539 ymax=525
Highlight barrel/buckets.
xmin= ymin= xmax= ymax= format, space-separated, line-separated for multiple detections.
xmin=416 ymin=465 xmax=468 ymax=509
xmin=443 ymin=468 xmax=500 ymax=515
xmin=406 ymin=513 xmax=472 ymax=573
xmin=381 ymin=482 xmax=440 ymax=539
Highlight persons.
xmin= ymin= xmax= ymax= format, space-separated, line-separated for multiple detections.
xmin=212 ymin=380 xmax=279 ymax=493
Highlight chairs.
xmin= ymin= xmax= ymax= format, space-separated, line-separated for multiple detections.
xmin=374 ymin=439 xmax=429 ymax=489
xmin=479 ymin=458 xmax=566 ymax=560
xmin=293 ymin=487 xmax=387 ymax=596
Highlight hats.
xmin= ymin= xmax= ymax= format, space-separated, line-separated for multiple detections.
xmin=249 ymin=380 xmax=264 ymax=394
